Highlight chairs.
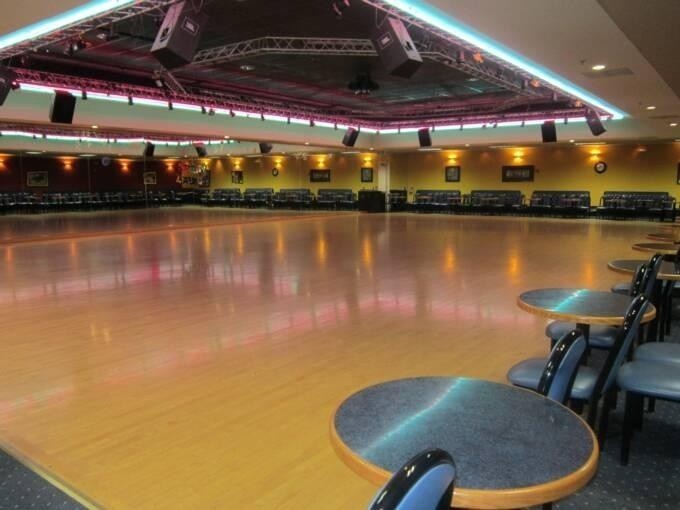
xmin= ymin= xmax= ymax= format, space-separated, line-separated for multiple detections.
xmin=507 ymin=293 xmax=655 ymax=457
xmin=635 ymin=337 xmax=680 ymax=365
xmin=616 ymin=358 xmax=678 ymax=467
xmin=367 ymin=446 xmax=458 ymax=509
xmin=519 ymin=328 xmax=591 ymax=413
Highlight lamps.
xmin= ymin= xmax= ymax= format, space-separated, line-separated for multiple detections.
xmin=63 ymin=37 xmax=86 ymax=58
xmin=347 ymin=67 xmax=380 ymax=94
xmin=80 ymin=88 xmax=339 ymax=130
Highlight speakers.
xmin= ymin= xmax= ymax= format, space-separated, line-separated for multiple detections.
xmin=48 ymin=89 xmax=76 ymax=123
xmin=541 ymin=120 xmax=557 ymax=142
xmin=193 ymin=143 xmax=207 ymax=157
xmin=368 ymin=17 xmax=423 ymax=78
xmin=145 ymin=143 xmax=155 ymax=157
xmin=418 ymin=128 xmax=431 ymax=147
xmin=259 ymin=143 xmax=273 ymax=153
xmin=342 ymin=127 xmax=359 ymax=147
xmin=0 ymin=79 xmax=11 ymax=106
xmin=585 ymin=111 xmax=606 ymax=136
xmin=150 ymin=1 xmax=209 ymax=70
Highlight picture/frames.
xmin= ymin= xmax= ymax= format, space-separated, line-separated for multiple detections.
xmin=361 ymin=167 xmax=373 ymax=182
xmin=231 ymin=170 xmax=243 ymax=184
xmin=501 ymin=164 xmax=535 ymax=183
xmin=197 ymin=170 xmax=211 ymax=187
xmin=309 ymin=168 xmax=331 ymax=182
xmin=141 ymin=170 xmax=158 ymax=185
xmin=26 ymin=169 xmax=50 ymax=188
xmin=446 ymin=166 xmax=461 ymax=181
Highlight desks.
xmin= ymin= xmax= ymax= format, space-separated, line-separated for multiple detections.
xmin=601 ymin=223 xmax=680 ymax=305
xmin=517 ymin=285 xmax=658 ymax=382
xmin=331 ymin=365 xmax=604 ymax=509
